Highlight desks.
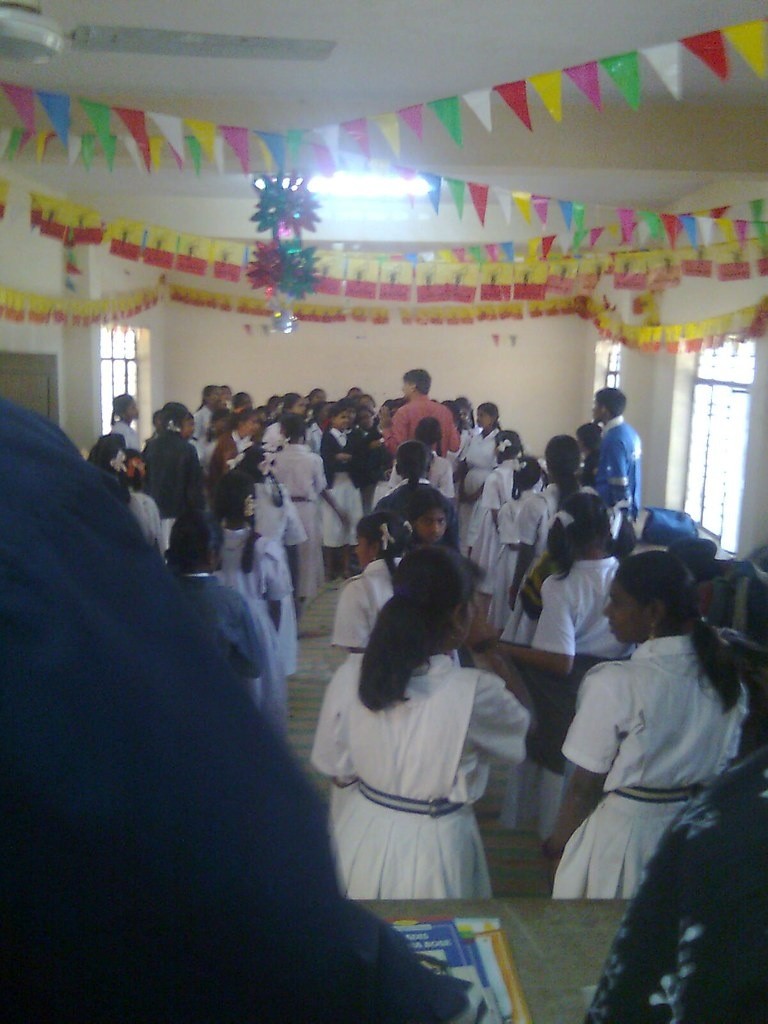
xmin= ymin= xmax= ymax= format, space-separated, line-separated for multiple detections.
xmin=355 ymin=898 xmax=629 ymax=1024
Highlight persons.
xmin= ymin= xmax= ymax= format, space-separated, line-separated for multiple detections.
xmin=0 ymin=394 xmax=495 ymax=1024
xmin=579 ymin=745 xmax=768 ymax=1024
xmin=85 ymin=369 xmax=768 ymax=901
